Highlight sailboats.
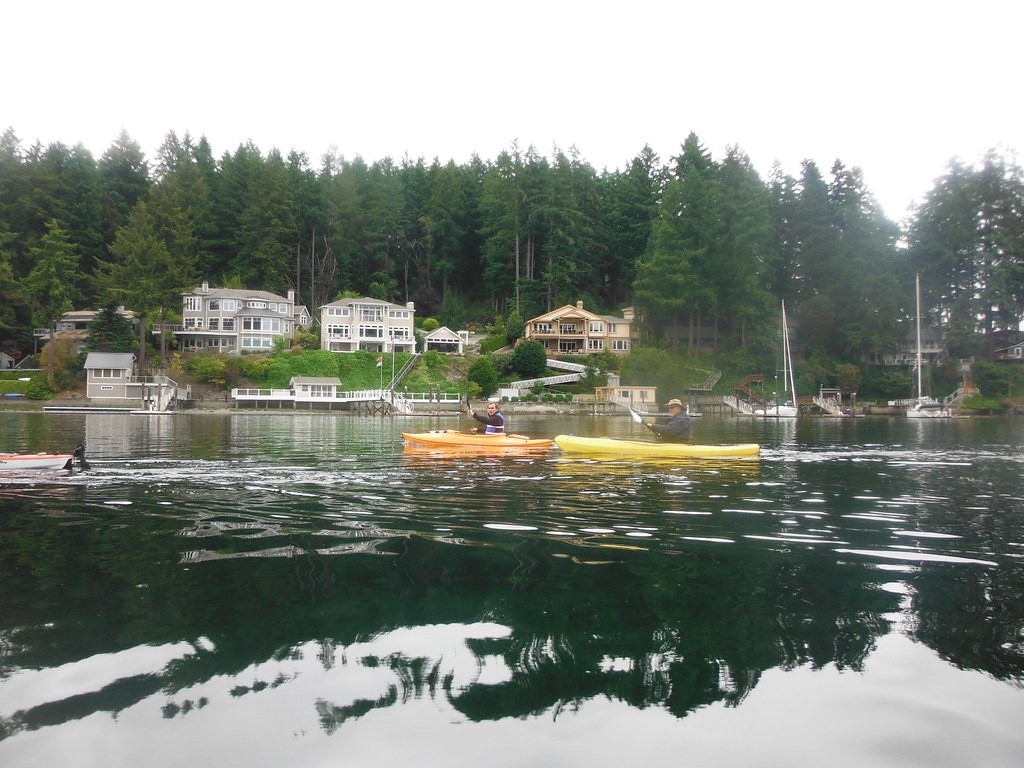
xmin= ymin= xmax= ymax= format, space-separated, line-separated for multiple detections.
xmin=905 ymin=272 xmax=953 ymax=418
xmin=754 ymin=298 xmax=799 ymax=417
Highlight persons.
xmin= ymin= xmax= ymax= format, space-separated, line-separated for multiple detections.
xmin=467 ymin=402 xmax=505 ymax=435
xmin=647 ymin=399 xmax=692 ymax=444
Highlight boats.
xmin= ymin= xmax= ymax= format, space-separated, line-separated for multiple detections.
xmin=553 ymin=434 xmax=762 ymax=461
xmin=402 ymin=430 xmax=555 ymax=452
xmin=0 ymin=453 xmax=75 ymax=472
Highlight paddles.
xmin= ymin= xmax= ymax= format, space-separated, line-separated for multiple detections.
xmin=465 ymin=395 xmax=477 ymax=435
xmin=628 ymin=405 xmax=662 ymax=439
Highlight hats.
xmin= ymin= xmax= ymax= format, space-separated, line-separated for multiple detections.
xmin=665 ymin=399 xmax=683 ymax=406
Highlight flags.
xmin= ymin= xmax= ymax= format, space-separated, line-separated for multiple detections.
xmin=376 ymin=362 xmax=382 ymax=368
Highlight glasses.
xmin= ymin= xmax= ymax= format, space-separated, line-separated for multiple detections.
xmin=667 ymin=406 xmax=679 ymax=410
